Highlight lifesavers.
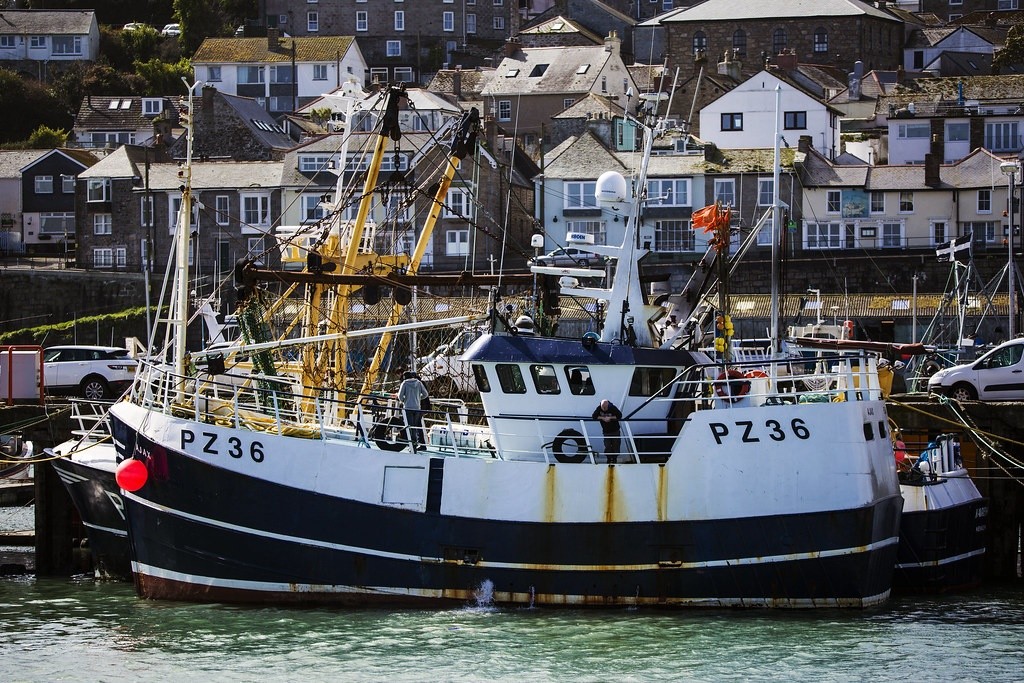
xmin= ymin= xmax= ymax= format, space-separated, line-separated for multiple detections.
xmin=745 ymin=370 xmax=768 ymax=377
xmin=553 ymin=429 xmax=588 ymax=463
xmin=374 ymin=417 xmax=409 ymax=451
xmin=716 ymin=370 xmax=749 ymax=403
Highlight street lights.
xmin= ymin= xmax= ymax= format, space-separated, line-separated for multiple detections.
xmin=999 ymin=162 xmax=1019 ymax=365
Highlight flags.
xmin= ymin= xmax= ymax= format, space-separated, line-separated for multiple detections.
xmin=692 ymin=203 xmax=730 ymax=249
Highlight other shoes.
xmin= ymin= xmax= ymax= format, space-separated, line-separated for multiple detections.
xmin=415 ymin=445 xmax=427 ymax=451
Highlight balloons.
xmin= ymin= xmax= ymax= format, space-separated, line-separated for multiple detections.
xmin=116 ymin=457 xmax=148 ymax=492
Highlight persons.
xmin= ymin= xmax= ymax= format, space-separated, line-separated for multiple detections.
xmin=399 ymin=371 xmax=433 ymax=452
xmin=592 ymin=400 xmax=622 ymax=463
xmin=968 ymin=332 xmax=984 ymax=368
xmin=894 ymin=440 xmax=911 ymax=483
xmin=912 ymin=443 xmax=936 ymax=485
xmin=495 ymin=297 xmax=505 ymax=331
xmin=58 ymin=352 xmax=68 ymax=360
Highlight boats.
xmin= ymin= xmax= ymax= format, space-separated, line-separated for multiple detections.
xmin=41 ymin=10 xmax=1011 ymax=620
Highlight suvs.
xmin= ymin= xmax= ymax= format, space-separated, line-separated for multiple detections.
xmin=35 ymin=345 xmax=139 ymax=401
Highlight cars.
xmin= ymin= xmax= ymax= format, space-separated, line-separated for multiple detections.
xmin=527 ymin=246 xmax=600 ymax=268
xmin=927 ymin=337 xmax=1024 ymax=402
xmin=121 ymin=22 xmax=159 ymax=36
xmin=161 ymin=23 xmax=182 ymax=37
